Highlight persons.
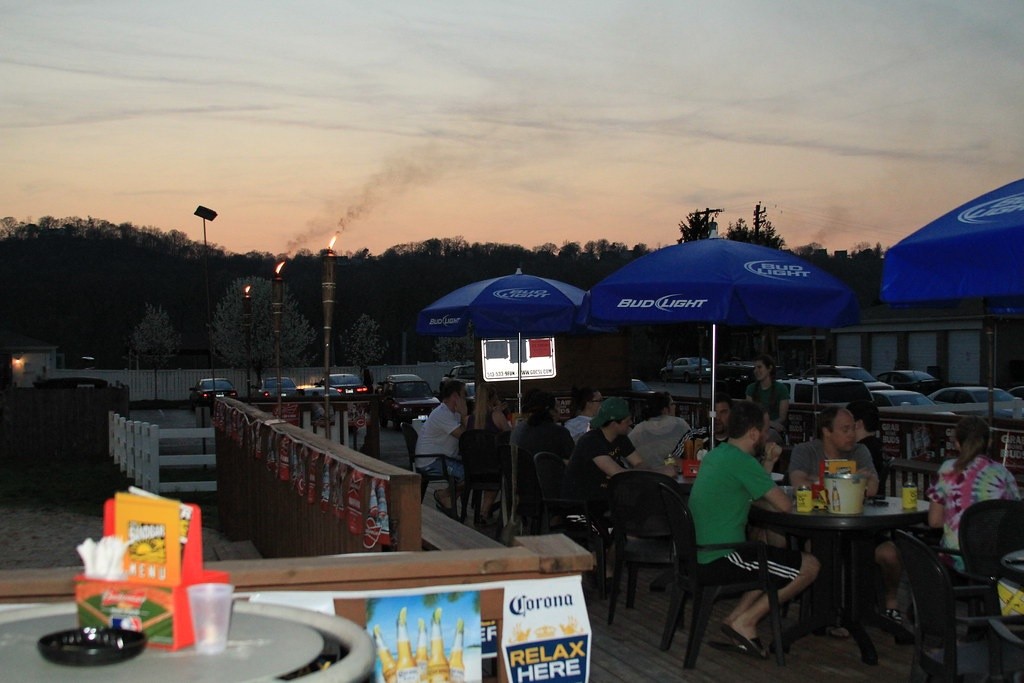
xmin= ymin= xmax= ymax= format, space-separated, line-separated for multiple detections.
xmin=618 ymin=392 xmax=692 ymax=469
xmin=294 ymin=389 xmax=335 ymax=422
xmin=927 ymin=415 xmax=1022 ymax=653
xmin=467 ymin=383 xmax=513 ymax=527
xmin=671 ymin=392 xmax=735 ymax=459
xmin=508 ymin=382 xmax=602 ymax=530
xmin=415 ymin=381 xmax=469 ymax=522
xmin=364 ymin=374 xmax=373 ymax=393
xmin=689 ymin=401 xmax=822 ymax=659
xmin=746 ymin=355 xmax=790 ymax=432
xmin=561 ymin=397 xmax=678 ymax=593
xmin=786 ymin=401 xmax=902 ymax=638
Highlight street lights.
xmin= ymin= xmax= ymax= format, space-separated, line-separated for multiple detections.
xmin=195 ymin=206 xmax=218 ymax=409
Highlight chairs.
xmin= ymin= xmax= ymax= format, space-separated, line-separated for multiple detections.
xmin=401 ymin=422 xmax=1023 ymax=682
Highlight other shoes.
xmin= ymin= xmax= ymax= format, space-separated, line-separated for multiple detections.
xmin=877 ymin=608 xmax=914 ymax=644
xmin=433 ymin=489 xmax=452 ymax=515
xmin=476 ymin=515 xmax=497 ymax=526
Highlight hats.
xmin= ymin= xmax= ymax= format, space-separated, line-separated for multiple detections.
xmin=590 ymin=398 xmax=629 ymax=427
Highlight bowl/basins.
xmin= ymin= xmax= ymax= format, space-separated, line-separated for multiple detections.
xmin=38 ymin=628 xmax=148 ymax=666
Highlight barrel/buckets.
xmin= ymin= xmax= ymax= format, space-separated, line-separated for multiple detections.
xmin=823 ymin=466 xmax=867 ymax=514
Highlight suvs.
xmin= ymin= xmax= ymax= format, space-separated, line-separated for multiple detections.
xmin=780 ymin=374 xmax=877 ymax=406
xmin=376 ymin=374 xmax=445 ymax=431
xmin=802 ymin=364 xmax=899 ymax=392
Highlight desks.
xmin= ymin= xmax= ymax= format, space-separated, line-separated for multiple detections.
xmin=650 ymin=466 xmax=786 ymax=602
xmin=749 ymin=494 xmax=930 ymax=666
xmin=0 ymin=603 xmax=376 ymax=683
xmin=1000 ymin=549 xmax=1024 ymax=585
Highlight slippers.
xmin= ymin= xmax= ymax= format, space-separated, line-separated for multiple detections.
xmin=722 ymin=625 xmax=767 ymax=658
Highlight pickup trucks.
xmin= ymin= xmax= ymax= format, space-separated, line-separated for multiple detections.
xmin=439 ymin=363 xmax=479 ymax=402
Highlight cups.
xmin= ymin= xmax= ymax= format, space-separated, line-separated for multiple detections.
xmin=797 ymin=485 xmax=812 ymax=512
xmin=901 ymin=481 xmax=918 ymax=508
xmin=186 ymin=583 xmax=234 ymax=653
xmin=664 ymin=454 xmax=674 ymax=464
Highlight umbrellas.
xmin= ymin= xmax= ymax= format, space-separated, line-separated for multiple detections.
xmin=416 ymin=269 xmax=618 ymax=413
xmin=575 ymin=240 xmax=862 ymax=451
xmin=878 ymin=177 xmax=1024 ymax=426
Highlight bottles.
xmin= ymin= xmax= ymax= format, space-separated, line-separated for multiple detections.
xmin=415 ymin=619 xmax=429 ymax=683
xmin=396 ymin=607 xmax=420 ymax=683
xmin=448 ymin=619 xmax=464 ymax=683
xmin=832 ymin=480 xmax=841 ymax=512
xmin=429 ymin=607 xmax=450 ymax=683
xmin=370 ymin=624 xmax=396 ymax=683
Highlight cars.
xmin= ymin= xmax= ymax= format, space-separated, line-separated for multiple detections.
xmin=926 ymin=384 xmax=1024 ymax=422
xmin=254 ymin=377 xmax=300 ymax=412
xmin=630 ymin=378 xmax=654 ymax=395
xmin=660 ymin=356 xmax=711 ymax=383
xmin=871 ymin=389 xmax=954 ymax=419
xmin=189 ymin=378 xmax=238 ymax=412
xmin=313 ymin=373 xmax=368 ymax=402
xmin=878 ymin=370 xmax=942 ymax=398
